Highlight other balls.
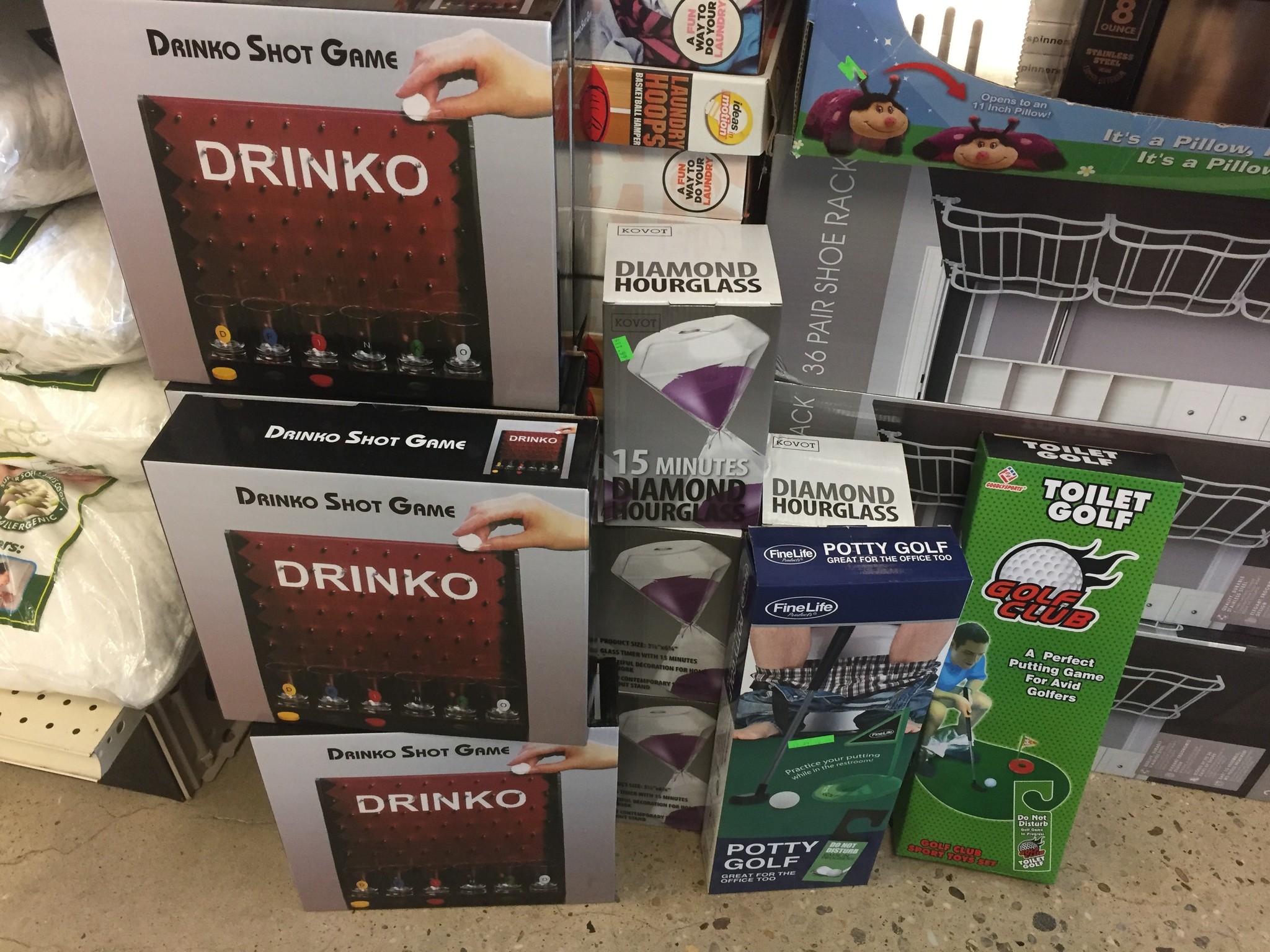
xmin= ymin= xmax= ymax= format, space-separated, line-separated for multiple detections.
xmin=458 ymin=533 xmax=482 ymax=551
xmin=511 ymin=763 xmax=531 ymax=774
xmin=403 ymin=93 xmax=432 ymax=121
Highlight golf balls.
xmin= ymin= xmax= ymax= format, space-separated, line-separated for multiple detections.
xmin=984 ymin=778 xmax=997 ymax=788
xmin=768 ymin=790 xmax=800 ymax=809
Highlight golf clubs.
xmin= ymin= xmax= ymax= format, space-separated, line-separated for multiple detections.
xmin=730 ymin=624 xmax=856 ymax=806
xmin=960 ymin=685 xmax=986 ymax=792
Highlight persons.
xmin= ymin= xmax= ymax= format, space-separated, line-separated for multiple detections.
xmin=397 ymin=30 xmax=551 ymax=124
xmin=915 ymin=623 xmax=992 ymax=777
xmin=508 ymin=737 xmax=619 ymax=774
xmin=732 ymin=618 xmax=959 ymax=741
xmin=454 ymin=492 xmax=588 ymax=554
